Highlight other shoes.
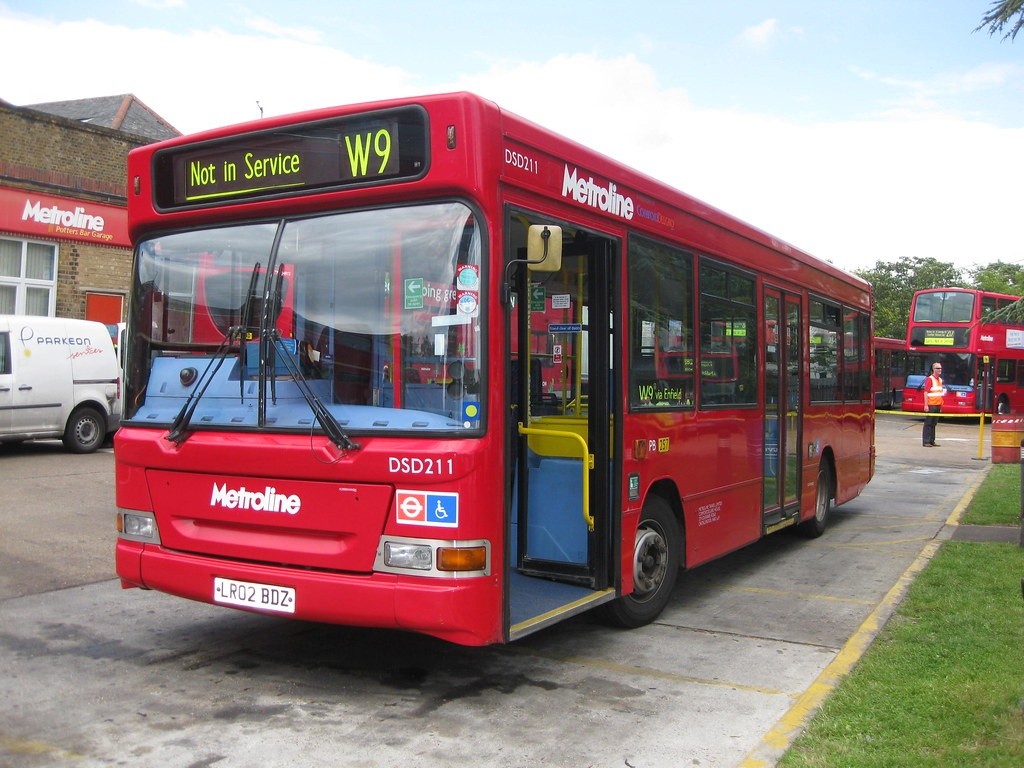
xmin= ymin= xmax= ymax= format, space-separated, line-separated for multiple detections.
xmin=923 ymin=444 xmax=933 ymax=447
xmin=932 ymin=442 xmax=940 ymax=446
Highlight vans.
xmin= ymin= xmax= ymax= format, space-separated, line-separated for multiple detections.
xmin=0 ymin=317 xmax=126 ymax=452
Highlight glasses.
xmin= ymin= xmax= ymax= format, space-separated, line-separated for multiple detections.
xmin=934 ymin=368 xmax=942 ymax=370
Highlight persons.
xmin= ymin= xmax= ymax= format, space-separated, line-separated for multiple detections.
xmin=922 ymin=363 xmax=943 ymax=447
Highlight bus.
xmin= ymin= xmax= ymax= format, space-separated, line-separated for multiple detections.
xmin=837 ymin=335 xmax=907 ymax=410
xmin=655 ymin=315 xmax=793 ymax=398
xmin=188 ymin=252 xmax=573 ymax=405
xmin=902 ymin=286 xmax=1023 ymax=418
xmin=111 ymin=92 xmax=874 ymax=648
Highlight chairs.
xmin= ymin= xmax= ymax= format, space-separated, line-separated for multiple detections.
xmin=634 ymin=354 xmax=837 ymax=404
xmin=512 ymin=360 xmax=543 ymax=404
